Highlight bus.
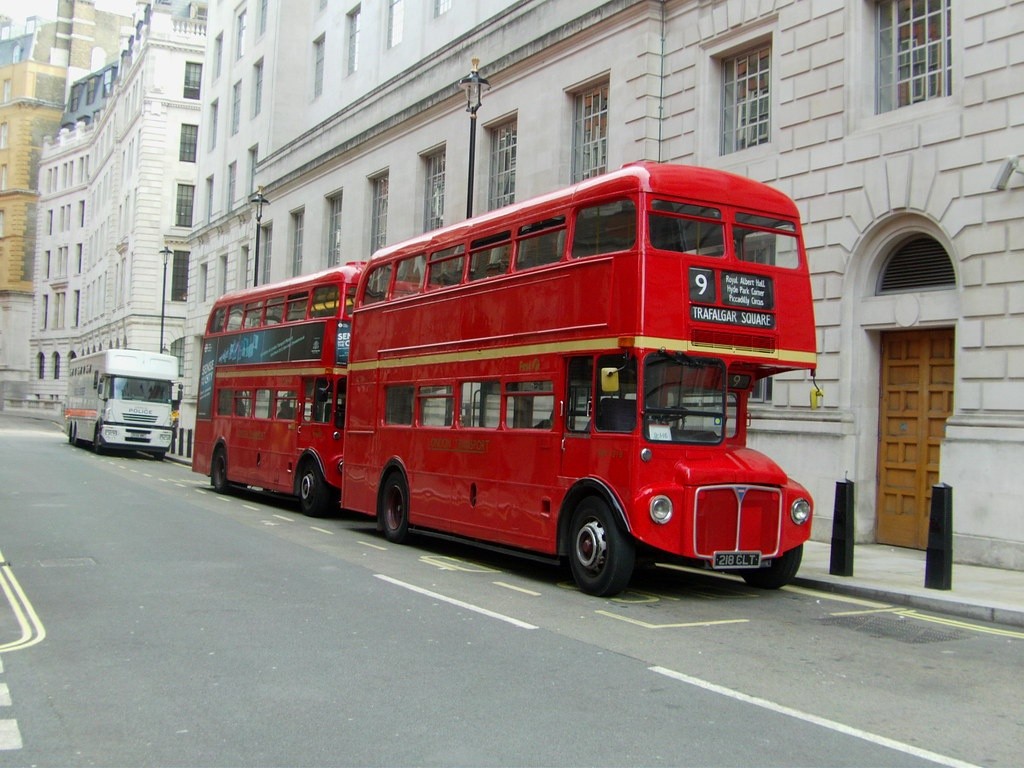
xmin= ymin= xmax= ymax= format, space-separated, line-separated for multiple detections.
xmin=189 ymin=259 xmax=370 ymax=513
xmin=339 ymin=159 xmax=824 ymax=598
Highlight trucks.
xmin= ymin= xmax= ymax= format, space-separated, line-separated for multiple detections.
xmin=62 ymin=346 xmax=185 ymax=461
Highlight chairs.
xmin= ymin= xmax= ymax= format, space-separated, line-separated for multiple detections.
xmin=585 ymin=398 xmax=636 ymax=432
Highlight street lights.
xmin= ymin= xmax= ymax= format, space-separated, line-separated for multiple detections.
xmin=456 ymin=53 xmax=494 ymax=220
xmin=249 ymin=182 xmax=270 ymax=289
xmin=158 ymin=244 xmax=171 ymax=356
